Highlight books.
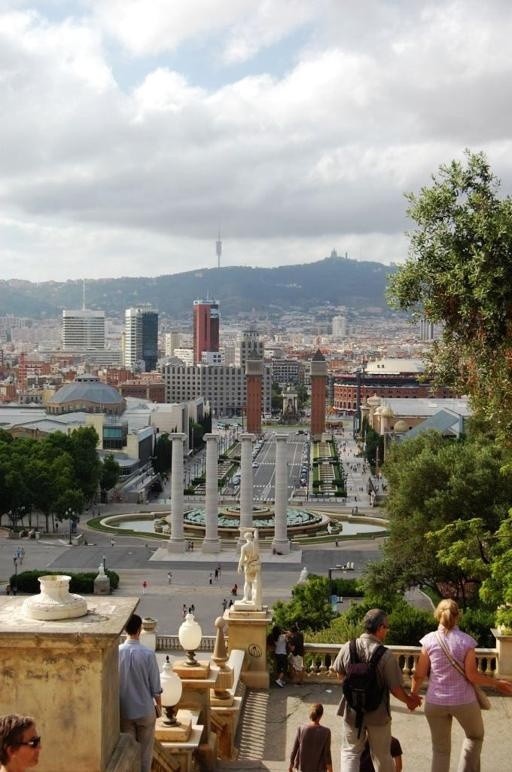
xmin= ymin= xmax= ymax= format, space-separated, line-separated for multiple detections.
xmin=153 ymin=703 xmax=163 ymax=718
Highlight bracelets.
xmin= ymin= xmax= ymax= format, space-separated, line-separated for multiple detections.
xmin=409 ymin=691 xmax=418 ymax=696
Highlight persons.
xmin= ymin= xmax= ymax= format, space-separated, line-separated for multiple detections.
xmin=237 ymin=527 xmax=263 ymax=604
xmin=209 ymin=573 xmax=213 ymax=584
xmin=168 ymin=572 xmax=173 ymax=583
xmin=214 ymin=567 xmax=218 ymax=578
xmin=142 ymin=581 xmax=147 ymax=594
xmin=188 ymin=607 xmax=192 ymax=613
xmin=183 ymin=604 xmax=187 ymax=617
xmin=12 ymin=545 xmax=26 ymax=565
xmin=286 ymin=624 xmax=306 ymax=686
xmin=222 ymin=599 xmax=228 ymax=611
xmin=191 ymin=603 xmax=195 ymax=611
xmin=269 ymin=625 xmax=292 ymax=688
xmin=232 ymin=584 xmax=238 ymax=596
xmin=407 ymin=595 xmax=512 ymax=772
xmin=0 ymin=711 xmax=44 ymax=772
xmin=288 ymin=703 xmax=334 ymax=772
xmin=330 ymin=607 xmax=419 ymax=772
xmin=117 ymin=613 xmax=164 ymax=771
xmin=229 ymin=600 xmax=233 ymax=607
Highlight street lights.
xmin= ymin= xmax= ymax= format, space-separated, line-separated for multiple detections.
xmin=65 ymin=507 xmax=75 ymax=545
xmin=13 ymin=545 xmax=24 ymax=586
xmin=328 ymin=561 xmax=354 ymax=595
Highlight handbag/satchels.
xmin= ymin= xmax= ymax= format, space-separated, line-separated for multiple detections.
xmin=472 ymin=686 xmax=492 ymax=710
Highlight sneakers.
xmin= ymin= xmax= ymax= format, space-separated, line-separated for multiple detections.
xmin=273 ymin=678 xmax=284 ymax=689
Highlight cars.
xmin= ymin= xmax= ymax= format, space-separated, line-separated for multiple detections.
xmin=296 ymin=431 xmax=307 ymax=435
xmin=252 ymin=440 xmax=263 ymax=458
xmin=232 ymin=475 xmax=240 ymax=485
xmin=300 ymin=442 xmax=309 ymax=486
xmin=252 ymin=462 xmax=259 ymax=468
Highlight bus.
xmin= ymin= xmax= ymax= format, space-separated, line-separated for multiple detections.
xmin=368 ymin=473 xmax=389 ymax=507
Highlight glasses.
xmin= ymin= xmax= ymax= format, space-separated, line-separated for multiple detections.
xmin=14 ymin=735 xmax=39 ymax=749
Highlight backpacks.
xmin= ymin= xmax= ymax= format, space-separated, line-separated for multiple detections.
xmin=341 ymin=636 xmax=388 ymax=716
